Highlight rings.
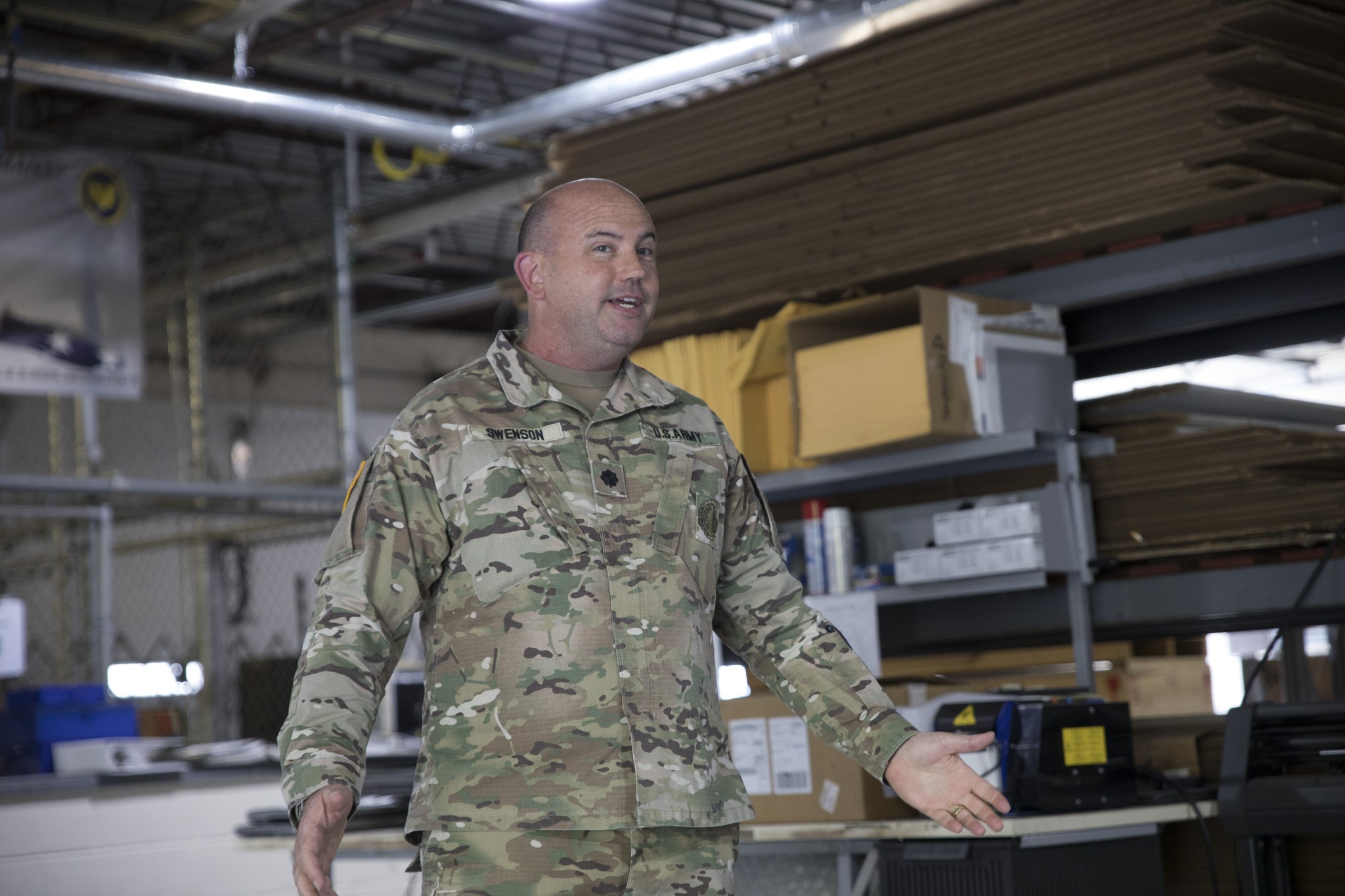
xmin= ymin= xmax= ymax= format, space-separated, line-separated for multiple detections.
xmin=952 ymin=804 xmax=965 ymax=817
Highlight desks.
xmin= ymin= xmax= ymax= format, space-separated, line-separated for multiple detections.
xmin=239 ymin=798 xmax=1221 ymax=896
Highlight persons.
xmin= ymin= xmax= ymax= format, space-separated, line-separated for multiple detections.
xmin=276 ymin=176 xmax=1012 ymax=896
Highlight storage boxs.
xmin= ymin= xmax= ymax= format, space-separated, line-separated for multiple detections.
xmin=785 ymin=286 xmax=1066 ymax=459
xmin=722 ymin=681 xmax=967 ymax=823
